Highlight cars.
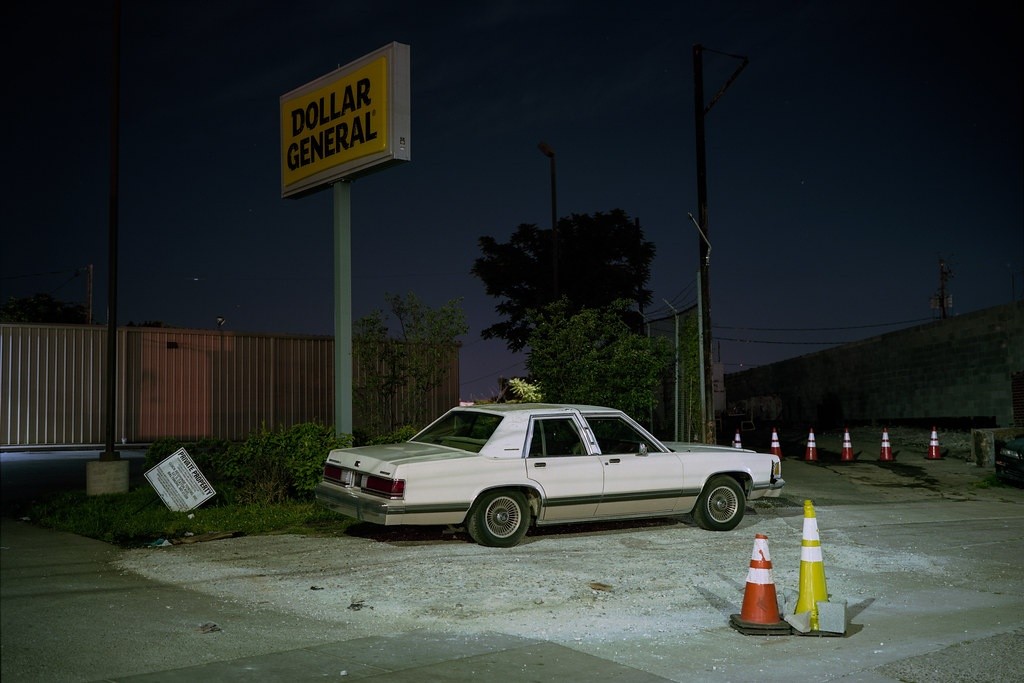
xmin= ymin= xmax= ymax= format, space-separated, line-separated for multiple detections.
xmin=994 ymin=433 xmax=1024 ymax=489
xmin=315 ymin=401 xmax=785 ymax=549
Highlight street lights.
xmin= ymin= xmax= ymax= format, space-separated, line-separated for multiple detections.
xmin=538 ymin=143 xmax=560 ymax=232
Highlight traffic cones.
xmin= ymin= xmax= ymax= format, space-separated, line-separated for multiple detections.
xmin=923 ymin=425 xmax=947 ymax=460
xmin=802 ymin=427 xmax=820 ymax=464
xmin=733 ymin=429 xmax=742 ymax=449
xmin=788 ymin=498 xmax=849 ymax=638
xmin=769 ymin=427 xmax=787 ymax=462
xmin=837 ymin=428 xmax=858 ymax=464
xmin=726 ymin=532 xmax=795 ymax=637
xmin=876 ymin=427 xmax=896 ymax=464
